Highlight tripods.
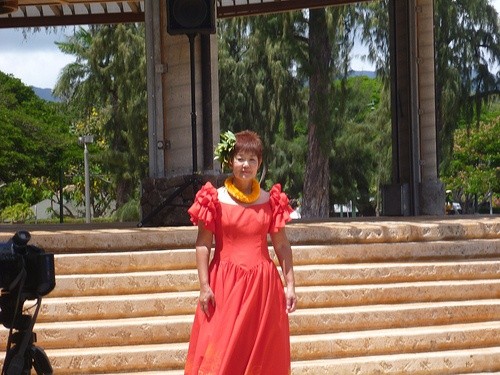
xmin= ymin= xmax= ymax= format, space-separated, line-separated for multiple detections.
xmin=138 ymin=36 xmax=210 ymax=226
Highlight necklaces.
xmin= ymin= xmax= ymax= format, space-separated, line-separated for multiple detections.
xmin=225 ymin=175 xmax=261 ymax=204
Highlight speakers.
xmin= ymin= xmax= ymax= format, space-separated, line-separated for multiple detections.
xmin=165 ymin=0 xmax=217 ymax=36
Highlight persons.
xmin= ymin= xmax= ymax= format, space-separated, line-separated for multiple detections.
xmin=184 ymin=128 xmax=300 ymax=375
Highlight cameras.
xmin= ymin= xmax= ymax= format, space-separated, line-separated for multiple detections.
xmin=0 ymin=232 xmax=56 ymax=300
